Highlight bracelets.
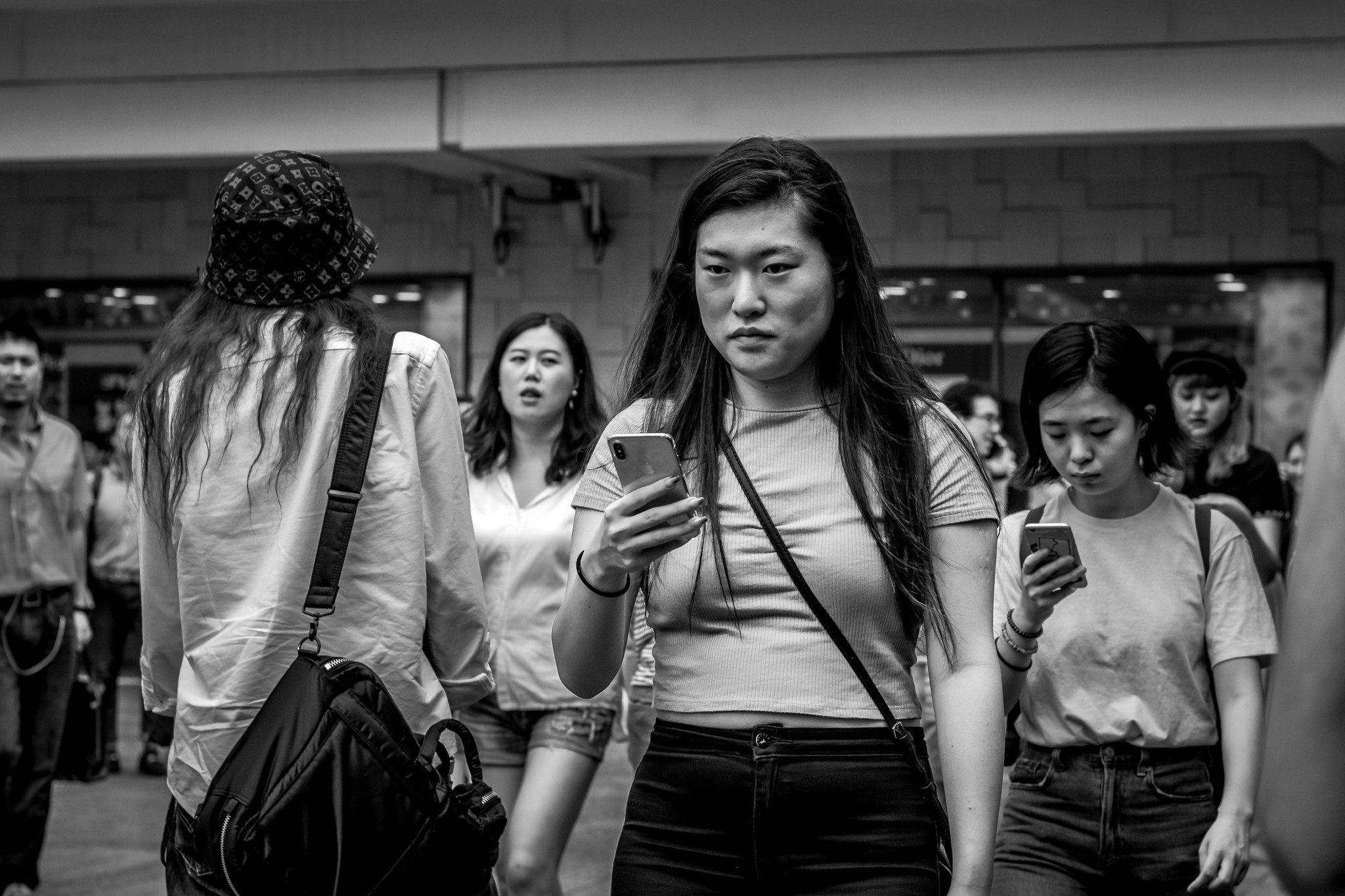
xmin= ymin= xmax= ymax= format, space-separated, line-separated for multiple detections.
xmin=576 ymin=550 xmax=632 ymax=596
xmin=1002 ymin=623 xmax=1038 ymax=654
xmin=1008 ymin=607 xmax=1044 ymax=638
xmin=995 ymin=637 xmax=1032 ymax=672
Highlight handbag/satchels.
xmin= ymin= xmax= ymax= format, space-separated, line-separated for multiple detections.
xmin=194 ymin=653 xmax=510 ymax=896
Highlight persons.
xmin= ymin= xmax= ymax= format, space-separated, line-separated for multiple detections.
xmin=126 ymin=151 xmax=486 ymax=896
xmin=1 ymin=331 xmax=95 ymax=896
xmin=618 ymin=584 xmax=655 ymax=781
xmin=1139 ymin=335 xmax=1288 ymax=631
xmin=990 ymin=316 xmax=1277 ymax=895
xmin=459 ymin=306 xmax=625 ymax=896
xmin=551 ymin=134 xmax=1005 ymax=896
xmin=942 ymin=380 xmax=1001 ymax=458
xmin=74 ymin=404 xmax=166 ymax=777
xmin=1278 ymin=432 xmax=1307 ymax=492
xmin=1254 ymin=325 xmax=1345 ymax=896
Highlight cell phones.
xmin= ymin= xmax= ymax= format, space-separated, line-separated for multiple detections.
xmin=607 ymin=433 xmax=698 ymax=532
xmin=1025 ymin=523 xmax=1088 ymax=589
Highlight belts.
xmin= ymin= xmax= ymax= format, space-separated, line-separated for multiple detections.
xmin=0 ymin=585 xmax=74 ymax=608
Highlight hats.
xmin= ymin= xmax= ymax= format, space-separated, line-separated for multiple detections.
xmin=200 ymin=150 xmax=378 ymax=305
xmin=1167 ymin=350 xmax=1247 ymax=388
